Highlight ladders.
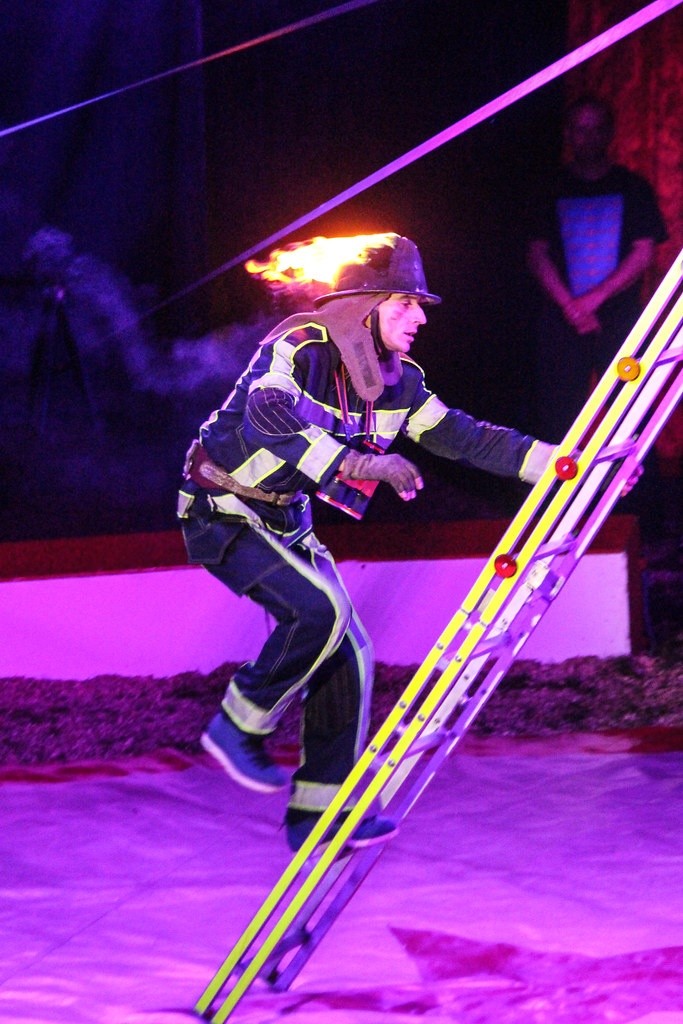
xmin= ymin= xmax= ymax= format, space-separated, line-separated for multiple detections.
xmin=191 ymin=251 xmax=683 ymax=1023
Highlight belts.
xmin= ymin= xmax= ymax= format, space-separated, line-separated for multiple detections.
xmin=185 ymin=445 xmax=303 ymax=506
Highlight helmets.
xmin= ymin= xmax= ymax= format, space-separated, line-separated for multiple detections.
xmin=312 ymin=233 xmax=440 ymax=305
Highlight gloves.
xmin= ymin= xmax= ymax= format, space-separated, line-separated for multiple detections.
xmin=342 ymin=452 xmax=426 ymax=501
xmin=520 ymin=434 xmax=643 ymax=495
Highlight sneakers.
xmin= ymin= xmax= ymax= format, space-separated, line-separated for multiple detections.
xmin=282 ymin=804 xmax=402 ymax=859
xmin=201 ymin=710 xmax=292 ymax=794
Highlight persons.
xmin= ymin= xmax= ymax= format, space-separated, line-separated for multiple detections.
xmin=521 ymin=92 xmax=669 ymax=545
xmin=177 ymin=236 xmax=644 ymax=859
